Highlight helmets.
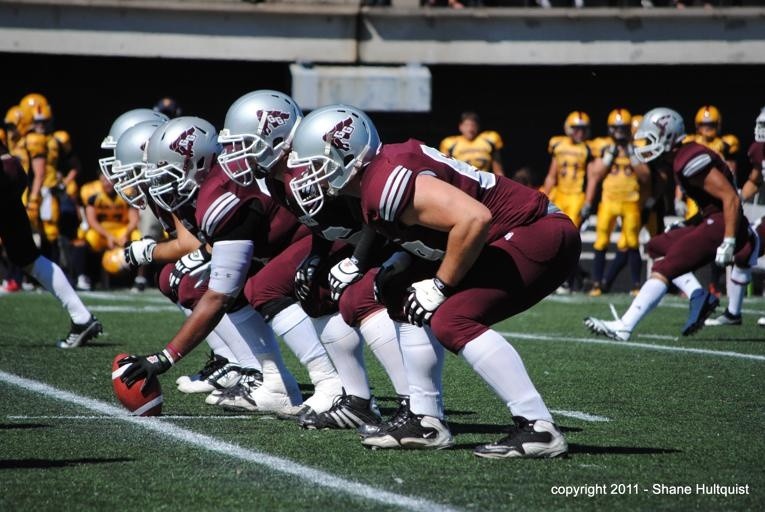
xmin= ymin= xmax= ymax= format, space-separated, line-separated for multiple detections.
xmin=4 ymin=94 xmax=78 ymax=201
xmin=99 ymin=89 xmax=383 ymax=219
xmin=102 ymin=247 xmax=126 ymax=274
xmin=565 ymin=105 xmax=723 ymax=164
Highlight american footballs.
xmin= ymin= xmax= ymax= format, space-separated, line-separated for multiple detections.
xmin=112 ymin=354 xmax=163 ymax=418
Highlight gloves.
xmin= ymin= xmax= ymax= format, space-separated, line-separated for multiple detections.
xmin=403 ymin=275 xmax=455 ymax=328
xmin=168 ymin=243 xmax=212 ymax=289
xmin=117 ymin=349 xmax=174 ymax=394
xmin=327 ymin=255 xmax=366 ymax=303
xmin=715 ymin=237 xmax=736 ymax=268
xmin=576 ymin=202 xmax=592 ymax=222
xmin=124 ymin=236 xmax=157 ymax=273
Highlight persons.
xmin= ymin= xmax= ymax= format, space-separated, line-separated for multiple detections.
xmin=0 ymin=91 xmax=764 ymax=463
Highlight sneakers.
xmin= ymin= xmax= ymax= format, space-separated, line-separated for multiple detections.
xmin=681 ymin=287 xmax=720 ymax=336
xmin=473 ymin=416 xmax=569 ymax=459
xmin=56 ymin=313 xmax=102 ymax=349
xmin=704 ymin=309 xmax=743 ymax=327
xmin=554 ymin=279 xmax=642 ymax=299
xmin=176 ymin=348 xmax=456 ymax=452
xmin=584 ymin=315 xmax=634 ymax=342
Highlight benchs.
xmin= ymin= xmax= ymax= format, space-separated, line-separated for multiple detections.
xmin=579 ymin=214 xmax=765 ymax=298
